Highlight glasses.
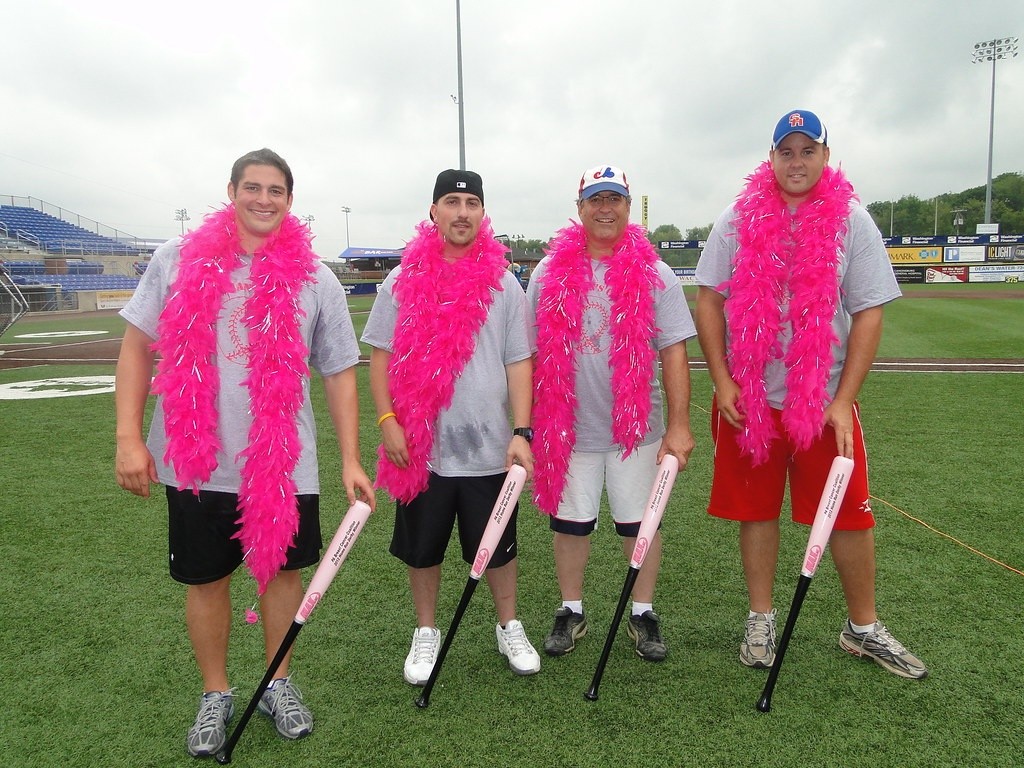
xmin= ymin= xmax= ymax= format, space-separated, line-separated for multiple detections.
xmin=587 ymin=194 xmax=625 ymax=205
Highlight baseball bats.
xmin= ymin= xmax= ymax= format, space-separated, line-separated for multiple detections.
xmin=412 ymin=463 xmax=528 ymax=708
xmin=755 ymin=455 xmax=856 ymax=712
xmin=582 ymin=452 xmax=680 ymax=702
xmin=214 ymin=498 xmax=373 ymax=765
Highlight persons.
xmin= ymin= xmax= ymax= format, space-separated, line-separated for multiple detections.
xmin=114 ymin=148 xmax=376 ymax=756
xmin=693 ymin=110 xmax=928 ymax=679
xmin=525 ymin=164 xmax=699 ymax=660
xmin=358 ymin=169 xmax=541 ymax=685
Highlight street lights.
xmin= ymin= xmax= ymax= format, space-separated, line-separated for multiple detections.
xmin=972 ymin=36 xmax=1018 ymax=223
xmin=174 ymin=208 xmax=191 ymax=235
xmin=302 ymin=215 xmax=315 ymax=236
xmin=342 ymin=206 xmax=351 ymax=248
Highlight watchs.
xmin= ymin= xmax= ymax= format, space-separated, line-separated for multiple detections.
xmin=514 ymin=427 xmax=534 ymax=442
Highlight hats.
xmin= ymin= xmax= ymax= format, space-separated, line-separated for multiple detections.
xmin=429 ymin=169 xmax=485 ymax=224
xmin=773 ymin=108 xmax=828 ymax=150
xmin=579 ymin=163 xmax=629 ymax=203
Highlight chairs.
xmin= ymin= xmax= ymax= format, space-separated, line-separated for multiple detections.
xmin=0 ymin=205 xmax=149 ymax=291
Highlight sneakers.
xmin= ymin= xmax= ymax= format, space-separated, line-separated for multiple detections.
xmin=626 ymin=609 xmax=667 ymax=662
xmin=543 ymin=605 xmax=588 ymax=656
xmin=403 ymin=626 xmax=442 ymax=687
xmin=496 ymin=619 xmax=541 ymax=676
xmin=186 ymin=685 xmax=240 ymax=758
xmin=838 ymin=617 xmax=929 ymax=680
xmin=739 ymin=607 xmax=777 ymax=669
xmin=257 ymin=678 xmax=314 ymax=740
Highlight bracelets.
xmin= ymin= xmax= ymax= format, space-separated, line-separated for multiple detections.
xmin=378 ymin=411 xmax=396 ymax=425
